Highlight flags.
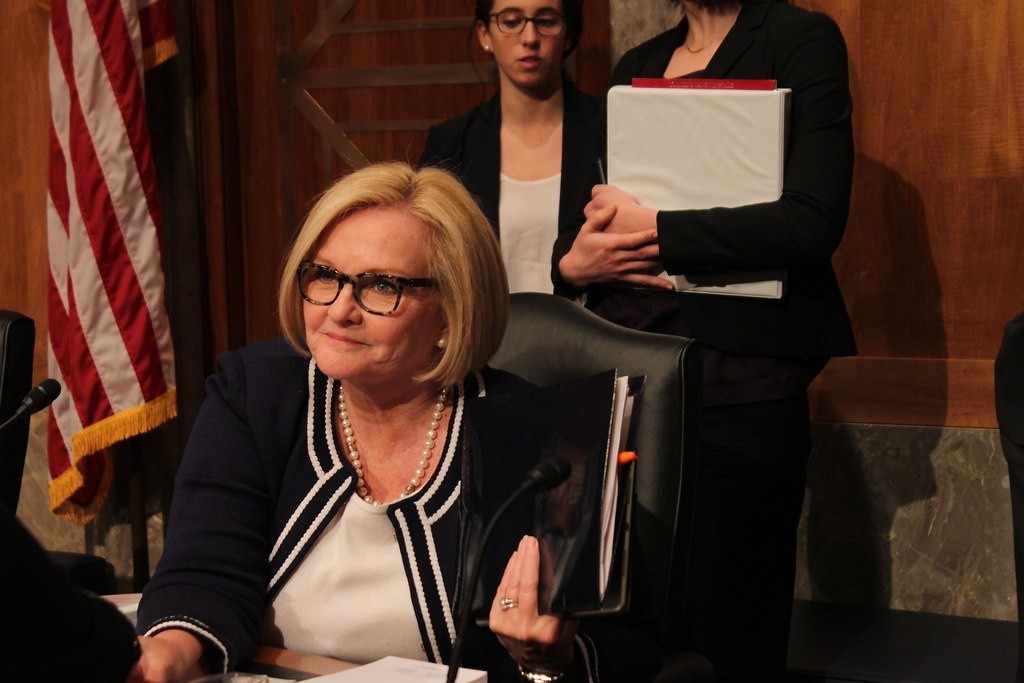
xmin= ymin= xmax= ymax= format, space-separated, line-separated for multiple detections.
xmin=50 ymin=0 xmax=177 ymax=525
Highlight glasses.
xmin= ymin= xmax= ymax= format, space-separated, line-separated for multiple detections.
xmin=297 ymin=259 xmax=437 ymax=316
xmin=487 ymin=10 xmax=567 ymax=37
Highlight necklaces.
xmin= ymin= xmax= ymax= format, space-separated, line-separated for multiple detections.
xmin=338 ymin=385 xmax=448 ymax=507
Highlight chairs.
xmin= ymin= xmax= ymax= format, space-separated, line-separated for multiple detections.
xmin=486 ymin=291 xmax=717 ymax=682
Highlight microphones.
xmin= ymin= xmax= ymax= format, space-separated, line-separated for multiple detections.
xmin=0 ymin=378 xmax=61 ymax=446
xmin=441 ymin=457 xmax=574 ymax=683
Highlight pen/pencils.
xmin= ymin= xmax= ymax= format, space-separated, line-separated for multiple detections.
xmin=596 ymin=157 xmax=606 ymax=185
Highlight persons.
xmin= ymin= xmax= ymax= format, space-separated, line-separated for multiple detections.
xmin=0 ymin=507 xmax=142 ymax=683
xmin=551 ymin=0 xmax=859 ymax=683
xmin=415 ymin=0 xmax=608 ymax=312
xmin=131 ymin=162 xmax=669 ymax=683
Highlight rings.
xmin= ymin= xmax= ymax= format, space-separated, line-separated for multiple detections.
xmin=501 ymin=599 xmax=517 ymax=610
